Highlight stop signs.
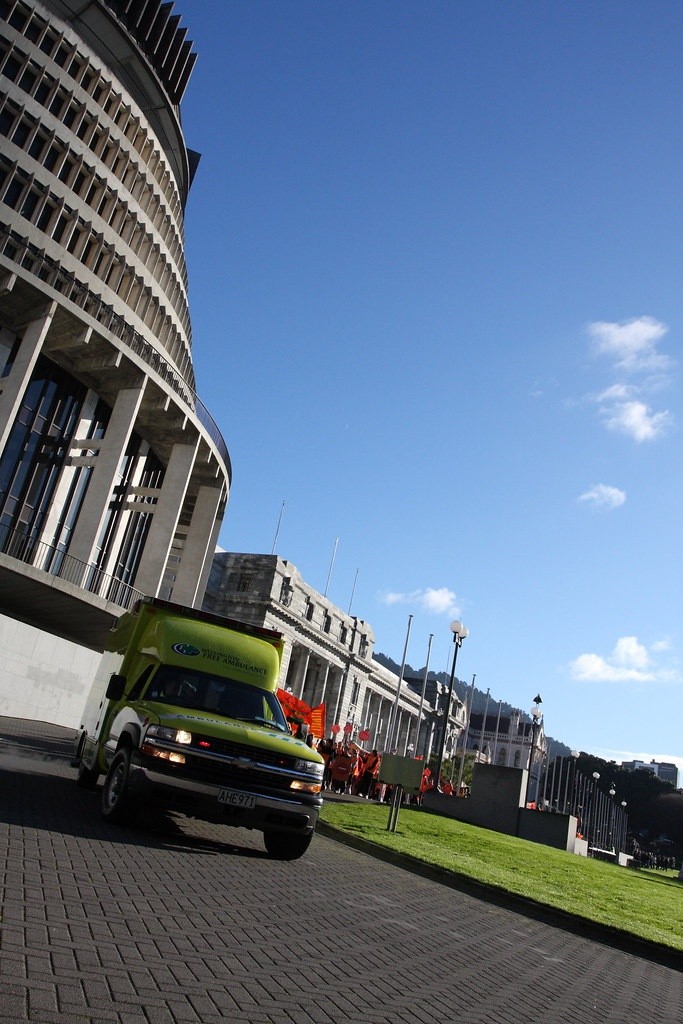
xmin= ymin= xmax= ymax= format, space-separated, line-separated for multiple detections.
xmin=335 ymin=763 xmax=348 ymax=776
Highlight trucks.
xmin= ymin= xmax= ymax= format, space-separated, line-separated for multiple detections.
xmin=70 ymin=596 xmax=327 ymax=860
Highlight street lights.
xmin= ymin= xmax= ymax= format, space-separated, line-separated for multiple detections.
xmin=607 ymin=789 xmax=616 ymax=850
xmin=429 ymin=620 xmax=470 ymax=793
xmin=569 ymin=750 xmax=580 ymax=814
xmin=524 ymin=708 xmax=543 ymax=807
xmin=591 ymin=772 xmax=601 ymax=843
xmin=620 ymin=800 xmax=627 ymax=850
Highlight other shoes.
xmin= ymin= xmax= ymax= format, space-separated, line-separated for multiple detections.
xmin=341 ymin=792 xmax=345 ymax=795
xmin=358 ymin=793 xmax=363 ymax=797
xmin=348 ymin=788 xmax=351 ymax=795
xmin=365 ymin=795 xmax=368 ymax=799
xmin=335 ymin=789 xmax=340 ymax=794
xmin=328 ymin=785 xmax=331 ymax=791
xmin=323 ymin=784 xmax=327 ymax=790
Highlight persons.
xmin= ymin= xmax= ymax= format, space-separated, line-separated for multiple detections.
xmin=631 ymin=839 xmax=676 ymax=872
xmin=574 ymin=812 xmax=615 ymax=862
xmin=401 ymin=763 xmax=470 ymax=807
xmin=538 ymin=802 xmax=560 ymax=813
xmin=151 ymin=673 xmax=191 ymax=704
xmin=287 ymin=722 xmax=395 ymax=805
